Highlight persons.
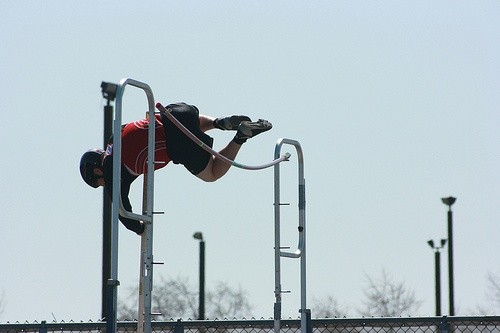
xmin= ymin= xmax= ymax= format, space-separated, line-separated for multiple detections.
xmin=80 ymin=102 xmax=273 ymax=236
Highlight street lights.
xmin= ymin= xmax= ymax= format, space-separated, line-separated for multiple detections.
xmin=99 ymin=81 xmax=119 ymax=323
xmin=428 ymin=238 xmax=447 ymax=316
xmin=441 ymin=196 xmax=457 ymax=317
xmin=192 ymin=231 xmax=206 ymax=320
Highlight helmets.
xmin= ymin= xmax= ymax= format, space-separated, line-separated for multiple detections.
xmin=79 ymin=148 xmax=102 ymax=188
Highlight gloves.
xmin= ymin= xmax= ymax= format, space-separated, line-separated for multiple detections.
xmin=135 ymin=223 xmax=145 ymax=235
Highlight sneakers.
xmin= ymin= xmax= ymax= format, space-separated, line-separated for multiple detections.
xmin=217 ymin=115 xmax=252 ymax=130
xmin=236 ymin=119 xmax=273 ymax=139
